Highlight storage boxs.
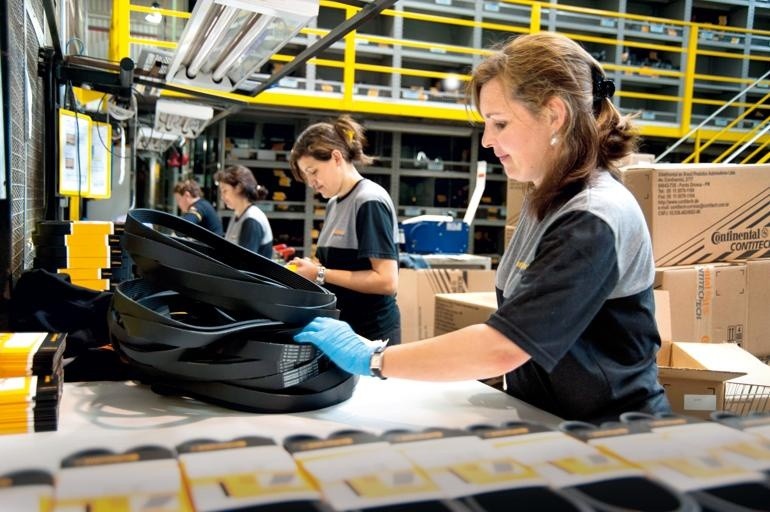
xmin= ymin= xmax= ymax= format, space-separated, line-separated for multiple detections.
xmin=730 ymin=260 xmax=769 ymax=358
xmin=610 ymin=153 xmax=656 ymax=175
xmin=432 ymin=292 xmax=504 ymax=389
xmin=648 ymin=287 xmax=673 ymax=371
xmin=399 ymin=266 xmax=494 ymax=346
xmin=653 ymin=363 xmax=748 ymax=416
xmin=504 ymin=175 xmax=537 ymax=255
xmin=651 ymin=262 xmax=748 ymax=357
xmin=622 ymin=164 xmax=769 ymax=268
xmin=670 ymin=341 xmax=769 ymax=417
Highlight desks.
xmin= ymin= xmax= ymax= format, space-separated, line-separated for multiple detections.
xmin=0 ymin=359 xmax=571 ymax=475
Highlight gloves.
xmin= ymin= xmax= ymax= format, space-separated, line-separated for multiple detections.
xmin=293 ymin=317 xmax=381 ymax=377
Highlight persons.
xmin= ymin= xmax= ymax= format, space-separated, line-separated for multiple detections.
xmin=173 ymin=178 xmax=222 ymax=238
xmin=214 ymin=166 xmax=273 ymax=261
xmin=293 ymin=31 xmax=675 ymax=420
xmin=286 ymin=115 xmax=402 ymax=345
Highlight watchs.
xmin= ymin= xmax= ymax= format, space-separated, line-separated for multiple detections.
xmin=315 ymin=267 xmax=323 ymax=287
xmin=370 ymin=348 xmax=387 ymax=381
